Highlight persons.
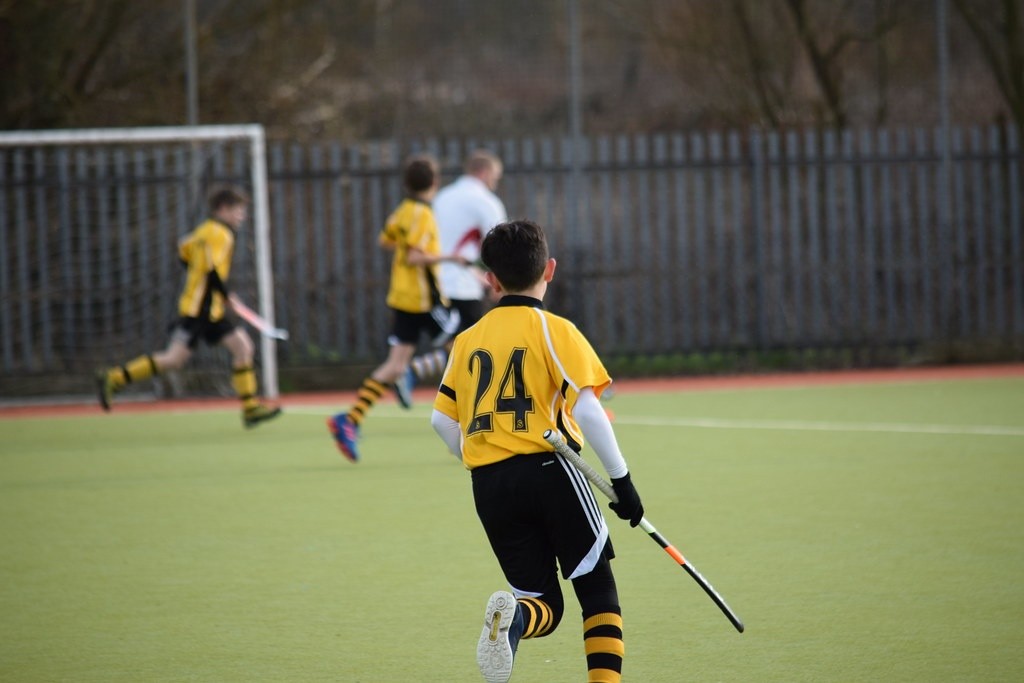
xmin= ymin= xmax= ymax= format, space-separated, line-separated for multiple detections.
xmin=328 ymin=160 xmax=460 ymax=463
xmin=432 ymin=218 xmax=645 ymax=683
xmin=433 ymin=151 xmax=515 ymax=333
xmin=95 ymin=184 xmax=282 ymax=429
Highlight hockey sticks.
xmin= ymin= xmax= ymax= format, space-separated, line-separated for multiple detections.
xmin=227 ymin=293 xmax=290 ymax=341
xmin=542 ymin=428 xmax=746 ymax=636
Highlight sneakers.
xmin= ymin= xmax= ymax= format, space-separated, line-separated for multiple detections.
xmin=328 ymin=412 xmax=359 ymax=462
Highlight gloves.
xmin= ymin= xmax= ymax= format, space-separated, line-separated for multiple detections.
xmin=609 ymin=470 xmax=644 ymax=528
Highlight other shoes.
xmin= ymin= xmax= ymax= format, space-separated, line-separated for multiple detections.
xmin=477 ymin=590 xmax=524 ymax=683
xmin=243 ymin=401 xmax=280 ymax=428
xmin=94 ymin=368 xmax=114 ymax=410
xmin=393 ymin=369 xmax=412 ymax=408
